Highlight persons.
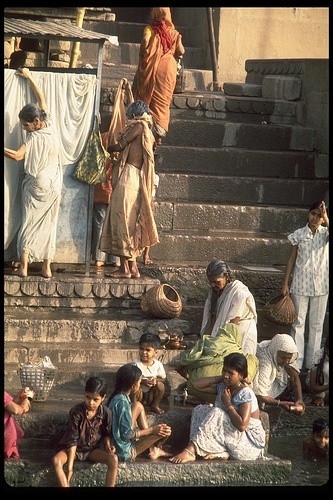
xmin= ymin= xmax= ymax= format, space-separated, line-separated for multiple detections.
xmin=92 ymin=78 xmax=159 ymax=279
xmin=281 ymin=201 xmax=329 ymax=408
xmin=127 ymin=333 xmax=166 ymax=414
xmin=4 ymin=67 xmax=62 ymax=278
xmin=51 ymin=377 xmax=118 ymax=487
xmin=105 ymin=365 xmax=173 ymax=461
xmin=132 ymin=8 xmax=184 ymax=145
xmin=254 ymin=333 xmax=305 ymax=417
xmin=4 ymin=388 xmax=34 ymax=459
xmin=169 ymin=353 xmax=265 ymax=464
xmin=303 ymin=417 xmax=329 ymax=459
xmin=176 ymin=261 xmax=257 ymax=397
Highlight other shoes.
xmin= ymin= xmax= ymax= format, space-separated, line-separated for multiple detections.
xmin=104 ymin=272 xmax=140 ymax=279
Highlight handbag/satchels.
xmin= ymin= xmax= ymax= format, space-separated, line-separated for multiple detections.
xmin=73 ymin=116 xmax=111 ymax=185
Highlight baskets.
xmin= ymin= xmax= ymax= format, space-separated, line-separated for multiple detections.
xmin=263 ymin=295 xmax=296 ymax=327
xmin=19 ymin=366 xmax=57 ymax=402
xmin=141 ymin=282 xmax=182 ymax=319
